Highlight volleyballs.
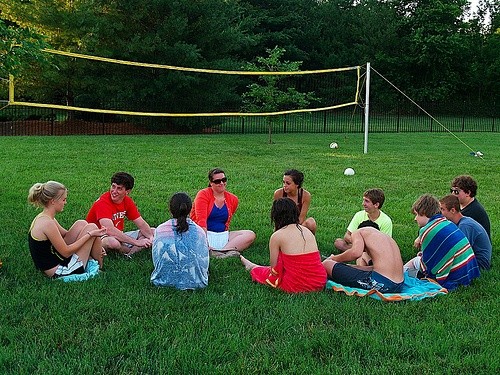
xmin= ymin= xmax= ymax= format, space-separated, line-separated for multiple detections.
xmin=329 ymin=142 xmax=339 ymax=148
xmin=343 ymin=167 xmax=355 ymax=175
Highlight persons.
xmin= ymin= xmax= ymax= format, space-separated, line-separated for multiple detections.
xmin=190 ymin=169 xmax=255 ymax=260
xmin=151 ymin=192 xmax=210 ymax=292
xmin=28 ymin=180 xmax=108 ymax=282
xmin=275 ymin=169 xmax=317 ymax=236
xmin=83 ymin=171 xmax=155 ymax=260
xmin=323 ymin=175 xmax=492 ymax=294
xmin=239 ymin=198 xmax=327 ymax=293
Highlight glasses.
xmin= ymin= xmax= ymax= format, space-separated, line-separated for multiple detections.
xmin=451 ymin=189 xmax=463 ymax=195
xmin=211 ymin=178 xmax=228 ymax=184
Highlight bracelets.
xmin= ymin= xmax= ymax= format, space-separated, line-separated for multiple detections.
xmin=88 ymin=232 xmax=91 ymax=237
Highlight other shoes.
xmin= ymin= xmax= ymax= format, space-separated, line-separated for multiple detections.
xmin=217 ymin=251 xmax=240 ymax=259
